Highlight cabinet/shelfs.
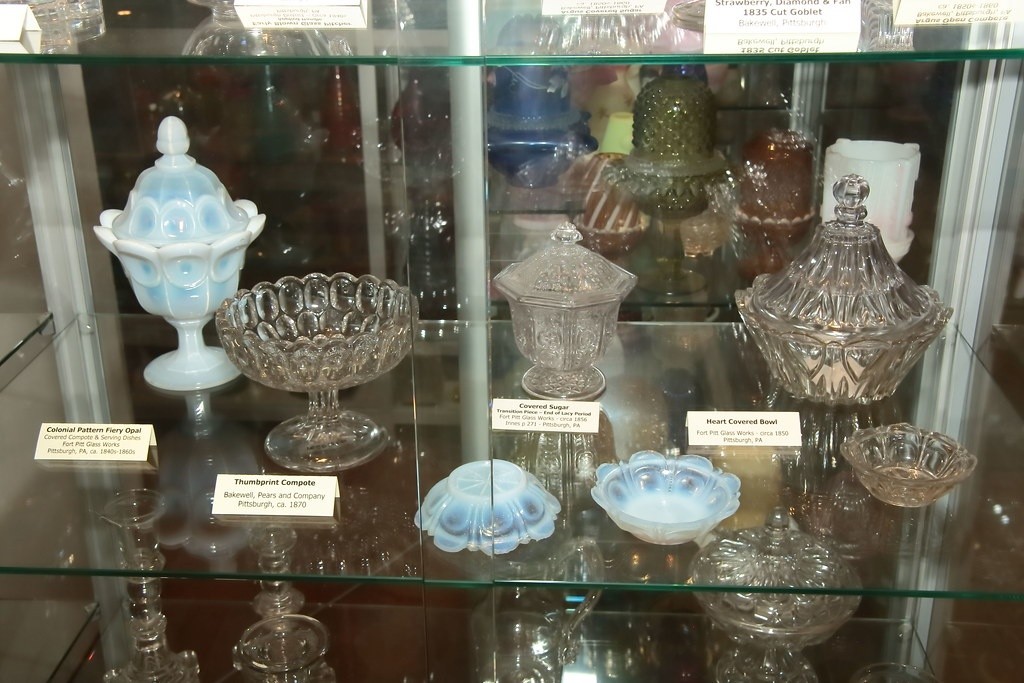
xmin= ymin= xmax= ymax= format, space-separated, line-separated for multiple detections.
xmin=0 ymin=0 xmax=1024 ymax=683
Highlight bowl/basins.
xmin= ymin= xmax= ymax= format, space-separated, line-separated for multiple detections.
xmin=588 ymin=448 xmax=742 ymax=547
xmin=838 ymin=421 xmax=978 ymax=508
xmin=412 ymin=459 xmax=563 ymax=557
